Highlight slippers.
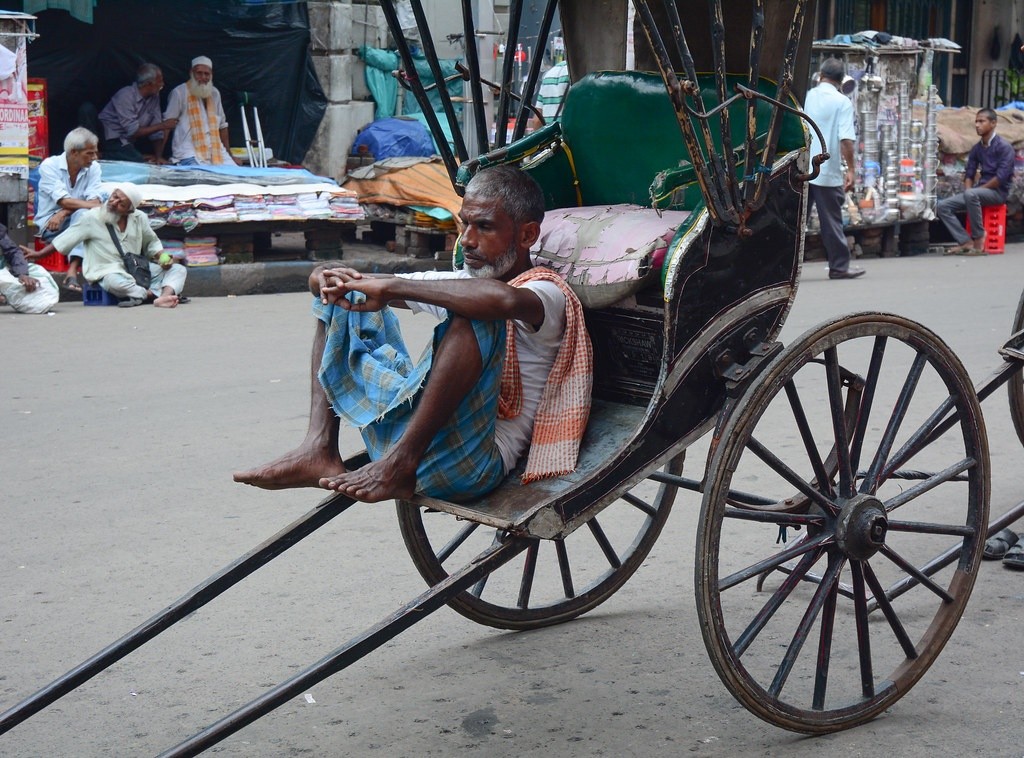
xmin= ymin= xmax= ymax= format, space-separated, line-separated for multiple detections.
xmin=981 ymin=527 xmax=1024 ymax=559
xmin=118 ymin=296 xmax=143 ymax=306
xmin=1001 ymin=537 xmax=1024 ymax=568
xmin=62 ymin=277 xmax=83 ymax=294
xmin=178 ymin=295 xmax=191 ymax=303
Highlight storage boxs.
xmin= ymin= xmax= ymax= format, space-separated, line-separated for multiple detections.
xmin=967 ymin=203 xmax=1008 ymax=254
xmin=34 ymin=236 xmax=78 ymax=273
xmin=82 ymin=284 xmax=119 ymax=306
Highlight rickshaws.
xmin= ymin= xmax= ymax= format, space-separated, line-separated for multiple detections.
xmin=2 ymin=0 xmax=1024 ymax=758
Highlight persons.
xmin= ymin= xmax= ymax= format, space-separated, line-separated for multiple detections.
xmin=936 ymin=108 xmax=1016 ymax=258
xmin=96 ymin=63 xmax=180 ymax=165
xmin=0 ymin=223 xmax=42 ymax=304
xmin=34 ymin=128 xmax=111 ymax=292
xmin=18 ymin=182 xmax=187 ymax=309
xmin=233 ymin=167 xmax=569 ymax=506
xmin=141 ymin=55 xmax=244 ymax=169
xmin=803 ymin=59 xmax=866 ymax=279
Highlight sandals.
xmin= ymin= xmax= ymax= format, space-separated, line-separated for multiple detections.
xmin=955 ymin=248 xmax=990 ymax=256
xmin=942 ymin=247 xmax=956 ymax=255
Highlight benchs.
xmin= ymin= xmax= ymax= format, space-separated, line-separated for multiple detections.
xmin=451 ymin=68 xmax=809 ymax=412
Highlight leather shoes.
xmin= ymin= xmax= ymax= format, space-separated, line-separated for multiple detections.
xmin=829 ymin=269 xmax=866 ymax=278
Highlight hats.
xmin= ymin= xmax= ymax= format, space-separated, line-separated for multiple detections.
xmin=192 ymin=56 xmax=213 ymax=70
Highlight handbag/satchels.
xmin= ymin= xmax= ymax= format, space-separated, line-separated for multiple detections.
xmin=125 ymin=252 xmax=152 ymax=290
